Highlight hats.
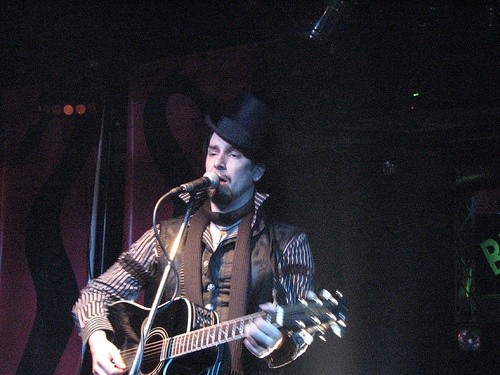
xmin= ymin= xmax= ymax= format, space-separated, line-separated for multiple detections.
xmin=205 ymin=97 xmax=279 ymax=171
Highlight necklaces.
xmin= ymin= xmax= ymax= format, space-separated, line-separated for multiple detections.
xmin=211 ymin=220 xmax=244 ymax=244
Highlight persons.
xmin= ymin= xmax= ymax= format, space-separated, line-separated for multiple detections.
xmin=72 ymin=95 xmax=316 ymax=375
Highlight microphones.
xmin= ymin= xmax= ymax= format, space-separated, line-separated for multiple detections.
xmin=170 ymin=171 xmax=220 ymax=194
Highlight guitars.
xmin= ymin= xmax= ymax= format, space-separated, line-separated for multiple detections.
xmin=78 ymin=288 xmax=349 ymax=375
